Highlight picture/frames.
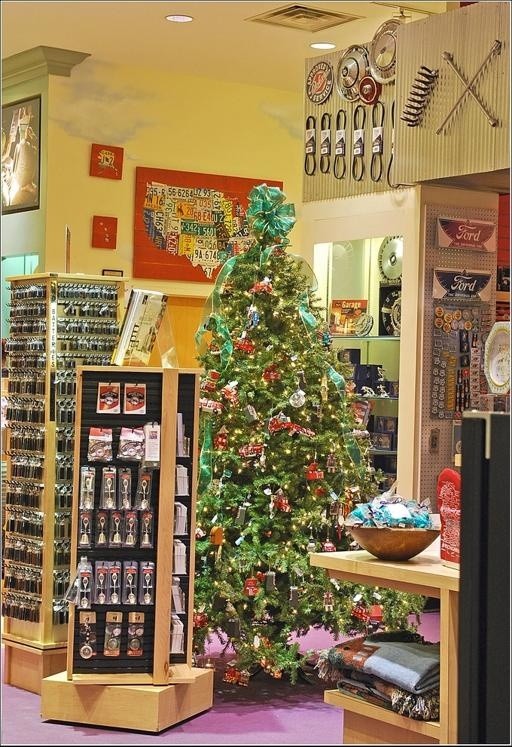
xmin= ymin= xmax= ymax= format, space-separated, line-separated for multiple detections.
xmin=0 ymin=95 xmax=41 ymax=216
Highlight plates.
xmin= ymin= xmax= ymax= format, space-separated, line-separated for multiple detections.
xmin=485 ymin=322 xmax=511 ymax=394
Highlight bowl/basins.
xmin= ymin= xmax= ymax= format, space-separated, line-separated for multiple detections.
xmin=344 ymin=526 xmax=441 ymax=562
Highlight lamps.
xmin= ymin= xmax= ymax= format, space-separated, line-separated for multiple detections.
xmin=164 ymin=15 xmax=193 ymax=24
xmin=308 ymin=43 xmax=336 ymax=51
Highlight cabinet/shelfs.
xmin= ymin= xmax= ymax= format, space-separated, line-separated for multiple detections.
xmin=313 ymin=228 xmax=420 ymax=492
xmin=40 ymin=362 xmax=215 ymax=732
xmin=305 ymin=410 xmax=510 ymax=746
xmin=5 ymin=273 xmax=128 ymax=695
